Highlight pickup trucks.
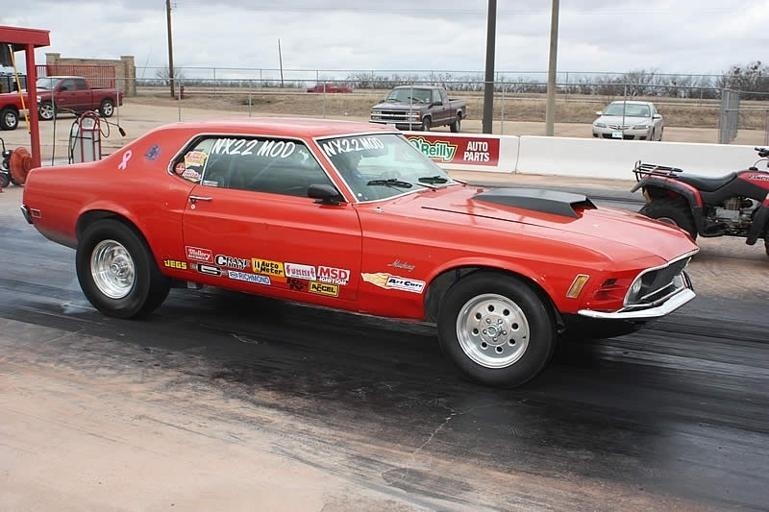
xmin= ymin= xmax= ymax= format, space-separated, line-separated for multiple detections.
xmin=0 ymin=76 xmax=123 ymax=130
xmin=369 ymin=86 xmax=467 ymax=132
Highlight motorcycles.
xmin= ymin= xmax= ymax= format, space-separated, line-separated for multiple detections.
xmin=630 ymin=147 xmax=768 ymax=258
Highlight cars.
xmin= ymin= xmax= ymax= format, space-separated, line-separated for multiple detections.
xmin=21 ymin=117 xmax=698 ymax=390
xmin=591 ymin=101 xmax=663 ymax=141
xmin=306 ymin=82 xmax=353 ymax=93
